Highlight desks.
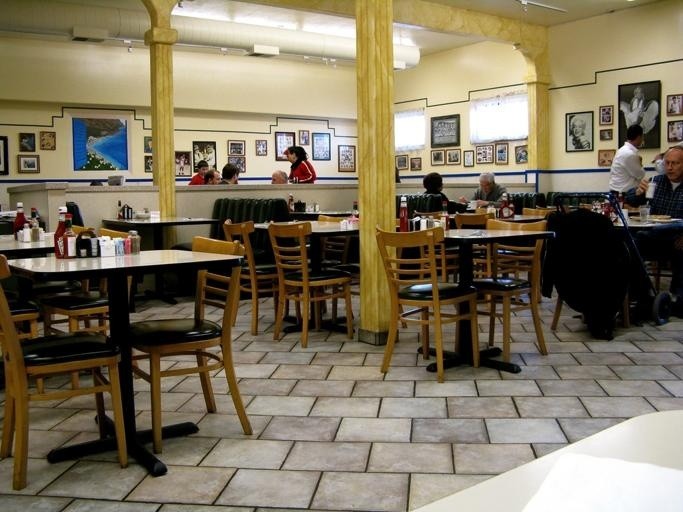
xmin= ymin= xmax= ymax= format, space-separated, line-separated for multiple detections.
xmin=6 ymin=249 xmax=247 ymax=478
xmin=1 ymin=226 xmax=100 ymax=252
xmin=251 ymin=218 xmax=360 ymax=336
xmin=606 ymin=207 xmax=682 ymax=308
xmin=416 ymin=227 xmax=558 ymax=373
xmin=101 ymin=212 xmax=222 ymax=230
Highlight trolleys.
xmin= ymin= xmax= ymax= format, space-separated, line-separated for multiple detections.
xmin=545 ymin=191 xmax=673 ymax=340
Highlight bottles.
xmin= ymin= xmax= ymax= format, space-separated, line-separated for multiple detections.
xmin=591 ymin=191 xmax=623 ymax=222
xmin=428 ymin=216 xmax=434 ymax=229
xmin=53 ymin=206 xmax=67 ymax=258
xmin=13 ymin=202 xmax=26 ymax=241
xmin=128 ymin=231 xmax=140 ymax=254
xmin=419 ymin=216 xmax=426 ymax=231
xmin=499 ymin=193 xmax=514 ymax=220
xmin=287 ymin=194 xmax=293 ymax=212
xmin=314 ymin=199 xmax=319 ymax=212
xmin=63 ymin=214 xmax=77 ymax=258
xmin=29 ymin=208 xmax=38 ymax=227
xmin=441 ymin=201 xmax=450 ymax=231
xmin=23 ymin=224 xmax=30 ymax=242
xmin=399 ymin=197 xmax=408 ymax=231
xmin=31 ymin=222 xmax=40 ymax=242
xmin=115 ymin=200 xmax=122 ymax=220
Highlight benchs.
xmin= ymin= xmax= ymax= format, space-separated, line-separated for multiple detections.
xmin=152 ymin=194 xmax=289 ymax=294
xmin=397 ymin=189 xmax=608 ymax=215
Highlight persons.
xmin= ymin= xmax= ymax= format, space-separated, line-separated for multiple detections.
xmin=193 ymin=145 xmax=203 ymax=166
xmin=144 ymin=139 xmax=152 ymax=153
xmin=609 ymin=123 xmax=648 ymax=201
xmin=568 ymin=116 xmax=590 ymax=150
xmin=146 ymin=157 xmax=152 ymax=171
xmin=270 ymin=171 xmax=288 ymax=184
xmin=623 ymin=145 xmax=683 ymax=318
xmin=603 ymin=115 xmax=610 ymax=122
xmin=202 ymin=169 xmax=221 ymax=185
xmin=186 ymin=159 xmax=209 ymax=186
xmin=669 ymin=96 xmax=680 ymax=113
xmin=654 ymin=153 xmax=666 ymax=176
xmin=670 ymin=122 xmax=682 ymax=141
xmin=458 ymin=172 xmax=509 ymax=209
xmin=216 ymin=162 xmax=239 ymax=184
xmin=620 ymin=84 xmax=658 ymax=144
xmin=516 ymin=149 xmax=527 ymax=162
xmin=420 ymin=171 xmax=467 ymax=216
xmin=175 ymin=154 xmax=185 ymax=176
xmin=282 ymin=146 xmax=316 ymax=184
xmin=203 ymin=145 xmax=215 ymax=169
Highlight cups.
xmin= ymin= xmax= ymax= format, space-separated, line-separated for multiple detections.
xmin=639 ymin=206 xmax=650 ymax=222
xmin=617 ymin=209 xmax=628 ymax=222
xmin=645 ymin=182 xmax=657 ymax=200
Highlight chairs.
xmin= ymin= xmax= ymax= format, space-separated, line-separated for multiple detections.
xmin=134 ymin=236 xmax=264 ymax=454
xmin=222 ymin=218 xmax=302 ymax=336
xmin=318 ymin=207 xmax=555 ymax=227
xmin=455 ymin=217 xmax=549 ymax=362
xmin=100 ymin=227 xmax=129 ymax=237
xmin=267 ymin=219 xmax=356 ymax=348
xmin=1 ymin=278 xmax=132 ymax=493
xmin=73 ymin=225 xmax=96 ymax=235
xmin=373 ymin=224 xmax=481 ymax=374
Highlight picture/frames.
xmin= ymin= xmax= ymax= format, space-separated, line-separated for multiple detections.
xmin=227 ymin=156 xmax=247 ymax=174
xmin=255 ymin=138 xmax=269 ymax=157
xmin=174 ymin=149 xmax=194 ymax=181
xmin=337 ymin=144 xmax=357 ymax=174
xmin=598 ymin=128 xmax=614 ymax=144
xmin=143 ymin=156 xmax=153 ymax=173
xmin=0 ymin=135 xmax=11 ymax=178
xmin=17 ymin=131 xmax=37 ymax=153
xmin=143 ymin=135 xmax=153 ymax=154
xmin=600 ymin=105 xmax=615 ymax=126
xmin=566 ymin=111 xmax=594 ymax=153
xmin=665 ymin=95 xmax=683 ymax=117
xmin=16 ymin=153 xmax=42 ymax=174
xmin=226 ymin=138 xmax=247 ymax=156
xmin=310 ymin=132 xmax=332 ymax=161
xmin=192 ymin=140 xmax=219 ymax=174
xmin=71 ymin=116 xmax=130 ymax=172
xmin=617 ymin=81 xmax=661 ymax=148
xmin=598 ymin=148 xmax=615 ymax=168
xmin=667 ymin=120 xmax=682 ymax=144
xmin=395 ymin=115 xmax=531 ymax=172
xmin=38 ymin=130 xmax=57 ymax=152
xmin=274 ymin=130 xmax=297 ymax=162
xmin=298 ymin=130 xmax=312 ymax=146
